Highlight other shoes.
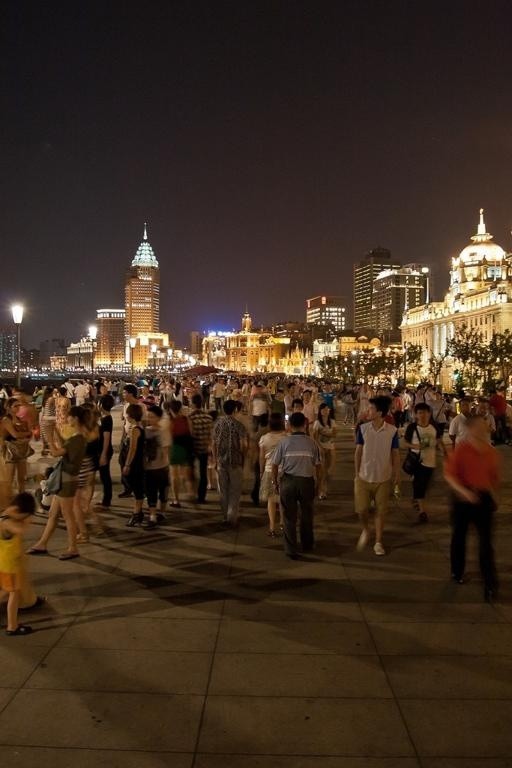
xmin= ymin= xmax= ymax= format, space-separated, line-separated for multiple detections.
xmin=373 ymin=542 xmax=385 ymax=557
xmin=357 ymin=528 xmax=367 ymax=550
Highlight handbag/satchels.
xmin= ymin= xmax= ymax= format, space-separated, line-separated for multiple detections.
xmin=38 ymin=467 xmax=63 ymax=497
xmin=2 ymin=439 xmax=36 ymax=464
xmin=401 ymin=451 xmax=423 ymax=478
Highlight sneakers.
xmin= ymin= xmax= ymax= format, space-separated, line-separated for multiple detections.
xmin=1 ymin=485 xmax=326 ymax=561
xmin=19 ymin=594 xmax=51 ymax=613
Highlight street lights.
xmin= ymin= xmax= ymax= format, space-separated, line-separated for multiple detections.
xmin=87 ymin=323 xmax=97 ymax=373
xmin=78 ymin=344 xmax=81 ymax=366
xmin=9 ymin=301 xmax=25 ymax=389
xmin=303 ymin=360 xmax=307 ymax=378
xmin=127 ymin=335 xmax=197 ymax=375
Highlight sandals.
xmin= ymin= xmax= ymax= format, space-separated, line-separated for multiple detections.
xmin=4 ymin=625 xmax=34 ymax=638
